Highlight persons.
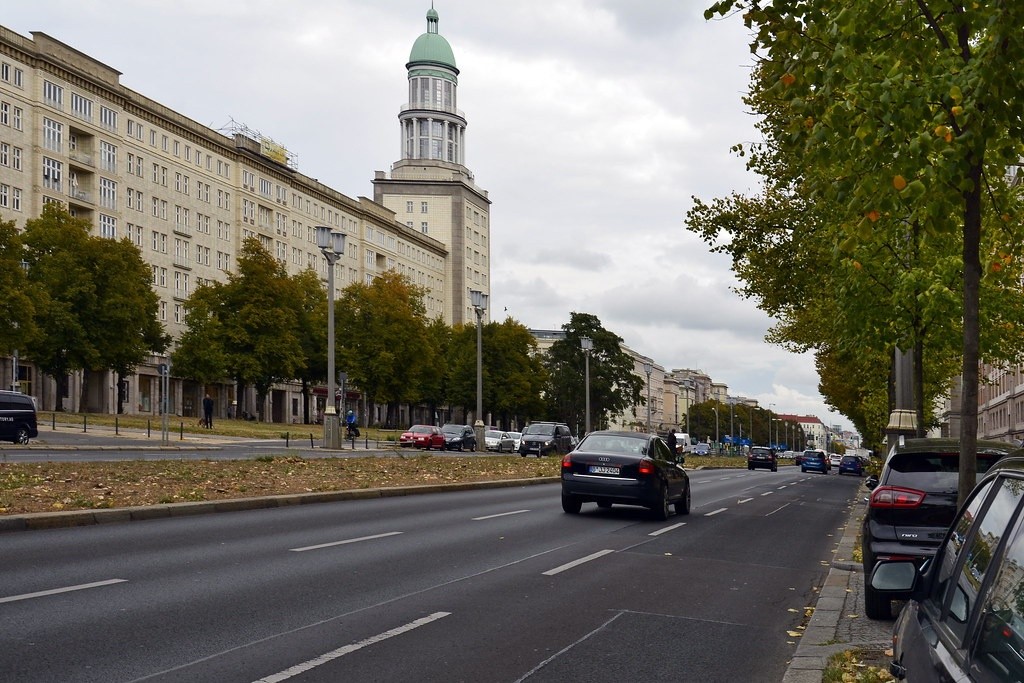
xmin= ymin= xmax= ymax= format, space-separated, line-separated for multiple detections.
xmin=201 ymin=392 xmax=214 ymax=430
xmin=345 ymin=410 xmax=361 ymax=439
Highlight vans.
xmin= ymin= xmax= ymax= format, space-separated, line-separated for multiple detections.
xmin=673 ymin=433 xmax=692 ymax=454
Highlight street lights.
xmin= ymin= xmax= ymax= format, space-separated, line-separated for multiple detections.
xmin=785 ymin=419 xmax=790 ymax=451
xmin=467 ymin=288 xmax=490 ymax=452
xmin=772 ymin=417 xmax=782 ymax=447
xmin=579 ymin=335 xmax=594 ymax=434
xmin=711 ymin=407 xmax=720 ymax=445
xmin=313 ymin=222 xmax=348 ymax=451
xmin=792 ymin=424 xmax=796 ymax=452
xmin=730 ymin=413 xmax=739 ymax=458
xmin=642 ymin=361 xmax=654 ymax=434
xmin=769 ymin=403 xmax=777 ymax=448
xmin=798 ymin=426 xmax=803 ymax=452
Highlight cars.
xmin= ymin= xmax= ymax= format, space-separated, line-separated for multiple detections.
xmin=794 ymin=454 xmax=803 ymax=466
xmin=0 ymin=390 xmax=39 ymax=446
xmin=484 ymin=430 xmax=523 ymax=454
xmin=694 ymin=443 xmax=712 ymax=457
xmin=438 ymin=422 xmax=477 ymax=451
xmin=837 ymin=455 xmax=863 ymax=476
xmin=862 ymin=441 xmax=1024 ymax=683
xmin=559 ymin=431 xmax=692 ymax=521
xmin=824 ymin=452 xmax=869 ymax=470
xmin=399 ymin=424 xmax=447 ymax=452
xmin=800 ymin=450 xmax=829 ymax=475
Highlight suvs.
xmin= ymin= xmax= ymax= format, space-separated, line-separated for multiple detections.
xmin=748 ymin=444 xmax=779 ymax=472
xmin=859 ymin=435 xmax=1024 ymax=622
xmin=519 ymin=421 xmax=572 ymax=459
